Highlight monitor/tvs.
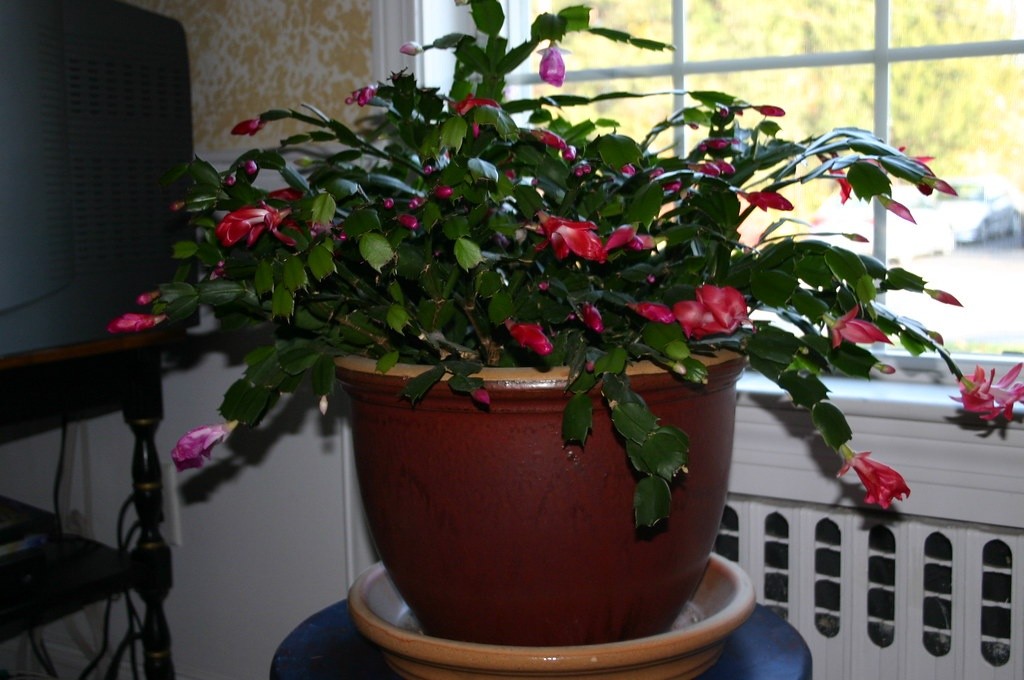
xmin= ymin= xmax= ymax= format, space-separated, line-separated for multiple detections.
xmin=0 ymin=0 xmax=195 ymax=357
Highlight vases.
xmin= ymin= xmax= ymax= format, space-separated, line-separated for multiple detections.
xmin=335 ymin=350 xmax=753 ymax=680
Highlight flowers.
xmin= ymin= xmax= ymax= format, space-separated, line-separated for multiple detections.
xmin=105 ymin=0 xmax=1024 ymax=530
